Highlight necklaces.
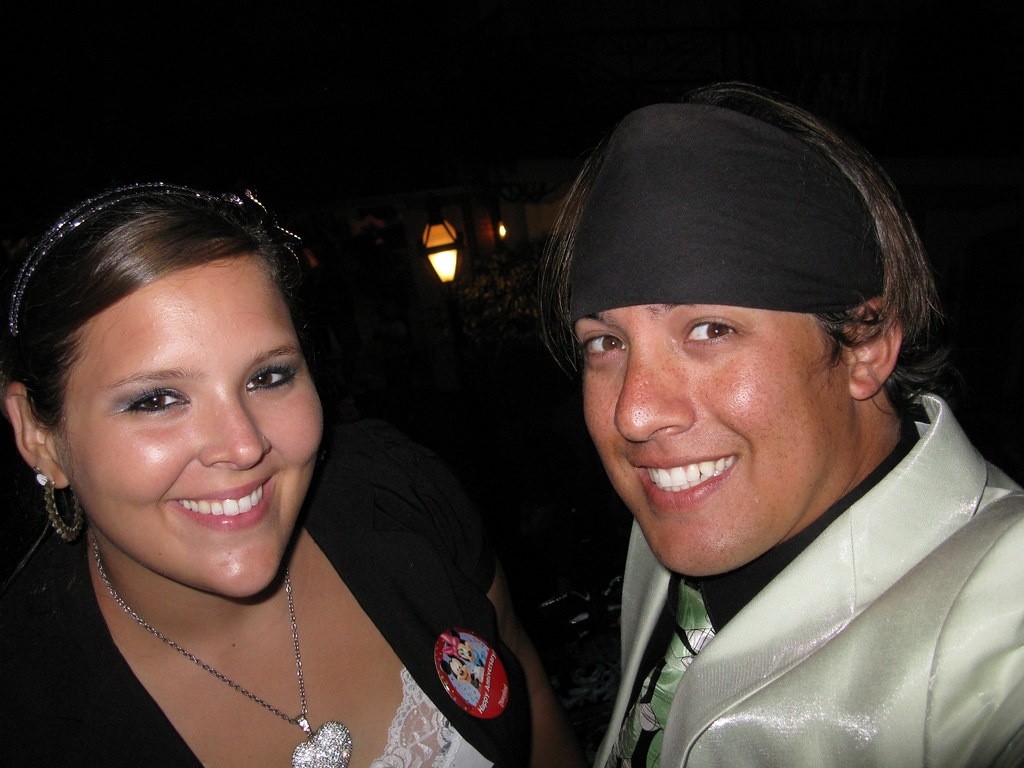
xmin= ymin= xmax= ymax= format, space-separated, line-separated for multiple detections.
xmin=92 ymin=533 xmax=353 ymax=768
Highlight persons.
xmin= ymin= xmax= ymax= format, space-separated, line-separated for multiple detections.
xmin=529 ymin=74 xmax=1024 ymax=768
xmin=0 ymin=176 xmax=577 ymax=767
